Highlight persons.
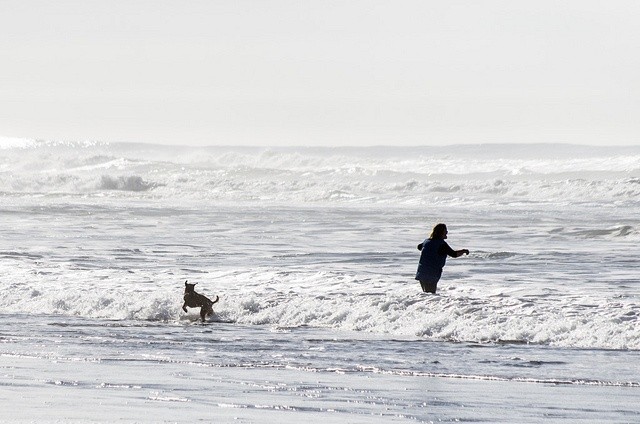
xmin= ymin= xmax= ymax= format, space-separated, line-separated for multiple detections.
xmin=415 ymin=224 xmax=469 ymax=293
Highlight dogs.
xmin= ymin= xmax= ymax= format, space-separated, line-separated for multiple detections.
xmin=182 ymin=280 xmax=219 ymax=322
